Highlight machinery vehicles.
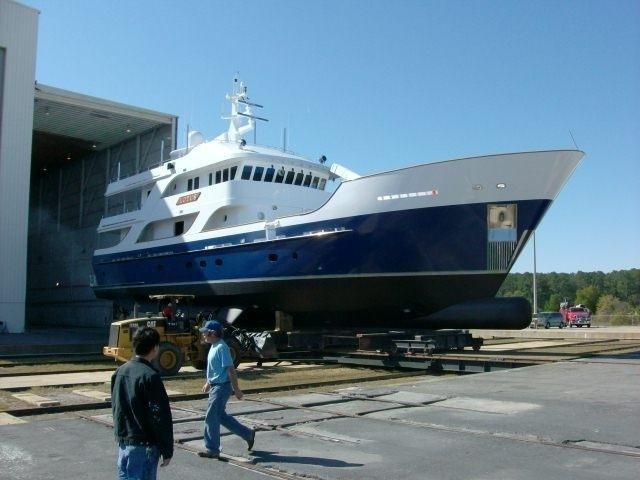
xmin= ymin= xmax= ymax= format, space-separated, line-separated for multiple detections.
xmin=101 ymin=293 xmax=242 ymax=375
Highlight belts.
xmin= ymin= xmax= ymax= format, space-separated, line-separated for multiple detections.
xmin=210 ymin=381 xmax=231 ymax=387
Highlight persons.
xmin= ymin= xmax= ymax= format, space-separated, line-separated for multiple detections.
xmin=110 ymin=324 xmax=174 ymax=480
xmin=197 ymin=320 xmax=256 ymax=459
xmin=162 ymin=302 xmax=175 ymax=321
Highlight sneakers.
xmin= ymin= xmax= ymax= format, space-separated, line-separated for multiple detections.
xmin=247 ymin=429 xmax=255 ymax=450
xmin=198 ymin=450 xmax=218 ymax=458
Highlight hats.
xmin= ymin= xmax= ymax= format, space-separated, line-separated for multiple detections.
xmin=199 ymin=320 xmax=222 ymax=333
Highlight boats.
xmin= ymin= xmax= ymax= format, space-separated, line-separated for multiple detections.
xmin=91 ymin=72 xmax=585 ymax=329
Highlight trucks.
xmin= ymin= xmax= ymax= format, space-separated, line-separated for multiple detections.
xmin=559 ymin=306 xmax=590 ymax=328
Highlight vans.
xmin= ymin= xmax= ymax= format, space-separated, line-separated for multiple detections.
xmin=538 ymin=312 xmax=564 ymax=329
